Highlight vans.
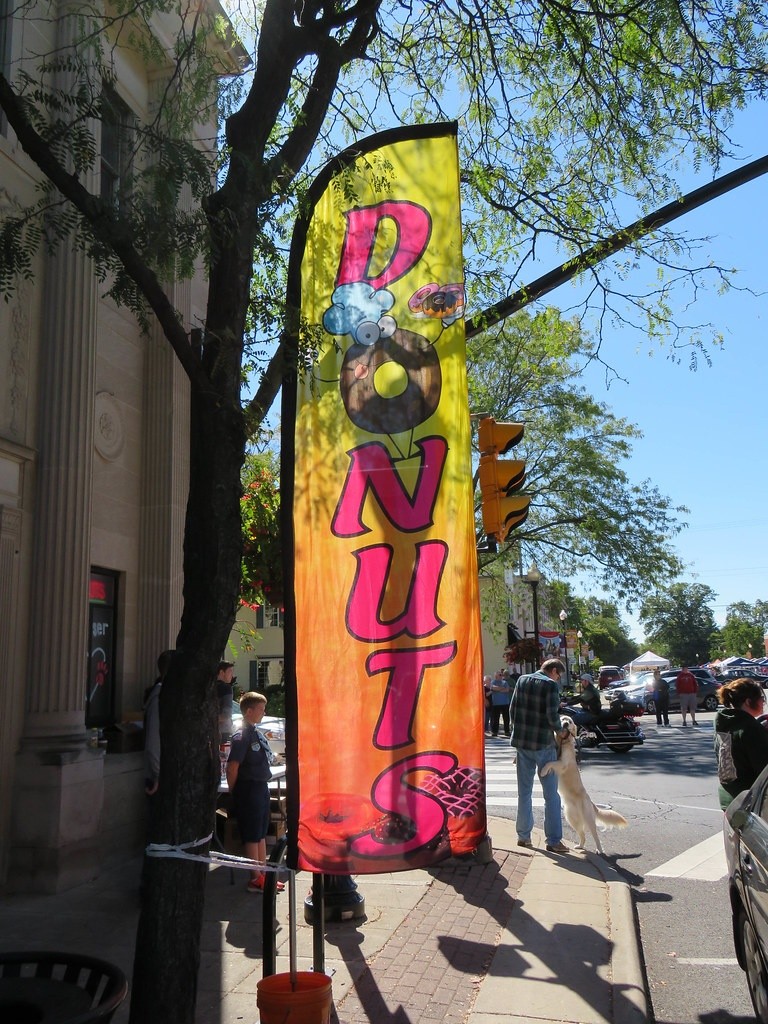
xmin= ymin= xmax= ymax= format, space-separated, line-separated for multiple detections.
xmin=599 ymin=669 xmax=620 ymax=690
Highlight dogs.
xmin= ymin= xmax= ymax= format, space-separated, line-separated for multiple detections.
xmin=512 ymin=714 xmax=628 ymax=853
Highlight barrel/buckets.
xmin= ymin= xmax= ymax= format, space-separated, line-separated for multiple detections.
xmin=256 ymin=971 xmax=332 ymax=1024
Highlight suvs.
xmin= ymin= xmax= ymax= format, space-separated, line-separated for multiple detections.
xmin=605 ymin=667 xmax=717 ymax=700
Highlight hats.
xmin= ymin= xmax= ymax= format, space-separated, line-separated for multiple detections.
xmin=680 ymin=664 xmax=688 ymax=667
xmin=582 ymin=674 xmax=594 ymax=685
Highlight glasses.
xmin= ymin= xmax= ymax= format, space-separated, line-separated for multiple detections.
xmin=557 ymin=671 xmax=561 ymax=679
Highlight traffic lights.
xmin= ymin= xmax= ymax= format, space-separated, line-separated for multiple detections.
xmin=477 ymin=417 xmax=530 ymax=540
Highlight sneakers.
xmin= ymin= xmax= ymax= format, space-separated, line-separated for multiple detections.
xmin=276 ymin=882 xmax=285 ymax=889
xmin=247 ymin=875 xmax=280 ymax=892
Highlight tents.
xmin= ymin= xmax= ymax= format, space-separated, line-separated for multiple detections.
xmin=623 ymin=651 xmax=671 ymax=677
xmin=701 ymin=656 xmax=768 ymax=674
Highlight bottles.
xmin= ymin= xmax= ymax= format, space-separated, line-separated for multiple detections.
xmin=90 ymin=728 xmax=98 ymax=747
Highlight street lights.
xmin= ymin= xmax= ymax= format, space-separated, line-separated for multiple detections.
xmin=559 ymin=609 xmax=568 ymax=685
xmin=577 ymin=630 xmax=582 ymax=656
xmin=528 ymin=569 xmax=541 ymax=671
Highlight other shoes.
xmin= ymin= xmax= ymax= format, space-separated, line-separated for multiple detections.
xmin=693 ymin=722 xmax=698 ymax=725
xmin=658 ymin=724 xmax=663 ymax=727
xmin=492 ymin=732 xmax=497 ymax=736
xmin=683 ymin=722 xmax=686 ymax=726
xmin=517 ymin=839 xmax=532 ymax=846
xmin=664 ymin=724 xmax=671 ymax=727
xmin=505 ymin=732 xmax=511 ymax=736
xmin=546 ymin=841 xmax=569 ymax=852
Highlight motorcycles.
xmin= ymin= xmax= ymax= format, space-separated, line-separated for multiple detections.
xmin=554 ymin=698 xmax=645 ymax=753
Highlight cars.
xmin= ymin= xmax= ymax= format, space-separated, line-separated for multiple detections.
xmin=626 ymin=676 xmax=720 ymax=713
xmin=712 ymin=669 xmax=768 ymax=688
xmin=218 ymin=713 xmax=286 ymax=801
xmin=608 ymin=671 xmax=655 ymax=688
xmin=722 ymin=762 xmax=768 ymax=1024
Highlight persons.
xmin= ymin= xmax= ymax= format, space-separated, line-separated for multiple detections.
xmin=713 ymin=677 xmax=768 ymax=812
xmin=509 ymin=659 xmax=569 ymax=852
xmin=217 ymin=661 xmax=235 ymax=750
xmin=653 ymin=671 xmax=671 ymax=727
xmin=225 ymin=692 xmax=285 ymax=895
xmin=559 ymin=674 xmax=601 ymax=725
xmin=484 ymin=667 xmax=520 ymax=738
xmin=675 ymin=666 xmax=698 ymax=726
xmin=145 ymin=649 xmax=175 ymax=803
